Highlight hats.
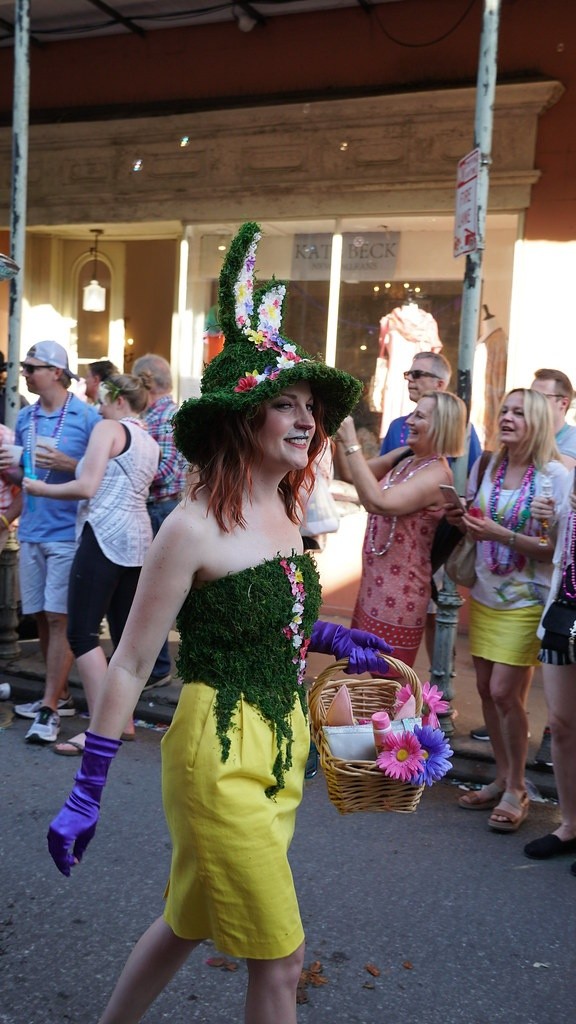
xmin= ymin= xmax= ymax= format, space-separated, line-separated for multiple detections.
xmin=30 ymin=341 xmax=80 ymax=382
xmin=174 ymin=223 xmax=364 ymax=459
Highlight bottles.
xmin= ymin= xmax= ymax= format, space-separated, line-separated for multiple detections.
xmin=371 ymin=711 xmax=396 ymax=759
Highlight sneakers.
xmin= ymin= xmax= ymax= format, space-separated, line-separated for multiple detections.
xmin=23 ymin=707 xmax=62 ymax=740
xmin=13 ymin=692 xmax=76 ymax=717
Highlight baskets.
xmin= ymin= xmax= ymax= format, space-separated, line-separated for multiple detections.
xmin=308 ymin=653 xmax=426 ymax=814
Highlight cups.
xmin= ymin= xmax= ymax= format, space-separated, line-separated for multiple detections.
xmin=35 ymin=435 xmax=56 ymax=455
xmin=1 ymin=444 xmax=24 ymax=467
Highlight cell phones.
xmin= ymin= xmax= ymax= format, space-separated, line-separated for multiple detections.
xmin=440 ymin=484 xmax=466 ymax=515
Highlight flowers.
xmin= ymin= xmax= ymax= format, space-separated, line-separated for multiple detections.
xmin=377 ymin=679 xmax=456 ymax=787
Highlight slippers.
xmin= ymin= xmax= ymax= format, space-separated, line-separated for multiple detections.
xmin=56 ymin=740 xmax=86 ymax=754
xmin=121 ymin=733 xmax=135 ymax=741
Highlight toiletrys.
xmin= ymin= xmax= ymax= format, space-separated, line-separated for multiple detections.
xmin=370 ymin=711 xmax=393 ymax=758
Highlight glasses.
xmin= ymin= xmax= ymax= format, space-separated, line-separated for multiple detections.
xmin=21 ymin=361 xmax=55 ymax=373
xmin=403 ymin=370 xmax=441 ymax=379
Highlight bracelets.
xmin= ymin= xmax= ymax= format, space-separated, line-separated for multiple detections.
xmin=0 ymin=513 xmax=10 ymax=527
xmin=345 ymin=444 xmax=362 ymax=456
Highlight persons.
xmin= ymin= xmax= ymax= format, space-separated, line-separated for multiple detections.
xmin=530 ymin=369 xmax=576 ymax=471
xmin=370 ymin=296 xmax=443 ymax=435
xmin=522 ymin=463 xmax=576 ymax=876
xmin=0 ymin=339 xmax=104 ymax=745
xmin=83 ymin=359 xmax=119 ymax=410
xmin=22 ymin=375 xmax=160 ymax=757
xmin=385 ymin=351 xmax=482 ymax=719
xmin=443 ymin=388 xmax=569 ymax=832
xmin=0 ymin=422 xmax=24 ymax=603
xmin=131 ymin=353 xmax=188 ymax=692
xmin=46 ymin=379 xmax=393 ymax=1024
xmin=335 ymin=389 xmax=468 ymax=686
xmin=0 ymin=350 xmax=29 ymax=426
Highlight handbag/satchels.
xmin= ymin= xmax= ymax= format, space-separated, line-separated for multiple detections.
xmin=444 ymin=528 xmax=476 ymax=589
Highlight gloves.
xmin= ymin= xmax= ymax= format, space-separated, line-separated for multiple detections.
xmin=306 ymin=620 xmax=395 ymax=676
xmin=46 ymin=729 xmax=123 ymax=876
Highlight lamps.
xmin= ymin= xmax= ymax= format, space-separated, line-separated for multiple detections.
xmin=84 ymin=230 xmax=105 ymax=312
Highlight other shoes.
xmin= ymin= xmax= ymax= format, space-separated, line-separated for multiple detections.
xmin=536 ymin=727 xmax=553 ymax=766
xmin=524 ymin=831 xmax=576 ymax=860
xmin=470 ymin=725 xmax=490 ymax=741
xmin=143 ymin=674 xmax=172 ymax=691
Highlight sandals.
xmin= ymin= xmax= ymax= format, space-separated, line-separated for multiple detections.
xmin=488 ymin=792 xmax=529 ymax=830
xmin=457 ymin=785 xmax=506 ymax=809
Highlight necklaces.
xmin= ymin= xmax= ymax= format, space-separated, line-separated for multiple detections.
xmin=122 ymin=414 xmax=145 ymax=427
xmin=561 ymin=504 xmax=576 ymax=599
xmin=27 ymin=390 xmax=74 ymax=479
xmin=483 ymin=449 xmax=533 ymax=576
xmin=370 ymin=457 xmax=436 ymax=555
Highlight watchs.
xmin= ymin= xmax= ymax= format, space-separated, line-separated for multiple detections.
xmin=508 ymin=532 xmax=517 ymax=548
